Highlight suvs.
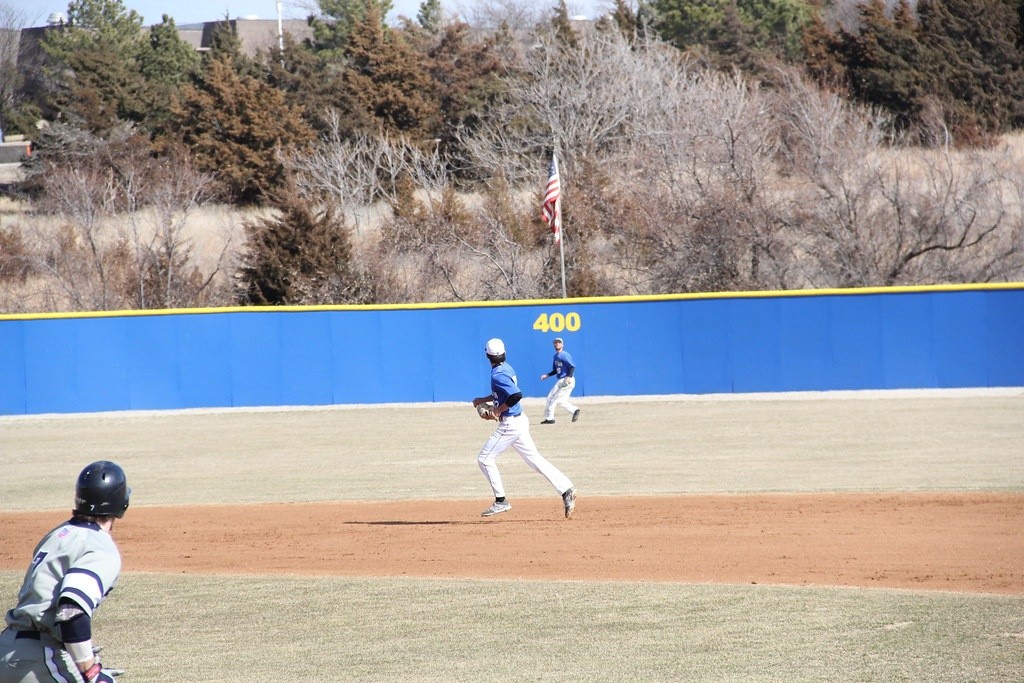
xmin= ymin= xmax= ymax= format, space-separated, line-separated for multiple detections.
xmin=0 ymin=140 xmax=39 ymax=193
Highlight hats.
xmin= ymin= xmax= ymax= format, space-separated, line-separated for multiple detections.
xmin=485 ymin=338 xmax=505 ymax=355
xmin=554 ymin=338 xmax=564 ymax=344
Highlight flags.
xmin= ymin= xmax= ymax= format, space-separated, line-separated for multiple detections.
xmin=541 ymin=154 xmax=560 ymax=244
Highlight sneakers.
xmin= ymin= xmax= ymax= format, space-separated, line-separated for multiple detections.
xmin=481 ymin=498 xmax=514 ymax=515
xmin=564 ymin=488 xmax=578 ymax=517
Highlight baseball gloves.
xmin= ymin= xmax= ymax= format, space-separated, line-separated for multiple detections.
xmin=558 ymin=380 xmax=571 ymax=388
xmin=477 ymin=399 xmax=499 ymax=422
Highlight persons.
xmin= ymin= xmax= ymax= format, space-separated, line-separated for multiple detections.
xmin=540 ymin=338 xmax=581 ymax=425
xmin=472 ymin=338 xmax=577 ymax=518
xmin=0 ymin=461 xmax=131 ymax=683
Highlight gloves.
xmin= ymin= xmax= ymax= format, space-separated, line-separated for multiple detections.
xmin=83 ymin=663 xmax=116 ymax=683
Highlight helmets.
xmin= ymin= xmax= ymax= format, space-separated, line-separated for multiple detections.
xmin=76 ymin=457 xmax=133 ymax=520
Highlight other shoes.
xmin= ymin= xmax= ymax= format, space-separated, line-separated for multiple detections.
xmin=540 ymin=419 xmax=556 ymax=424
xmin=572 ymin=409 xmax=580 ymax=423
